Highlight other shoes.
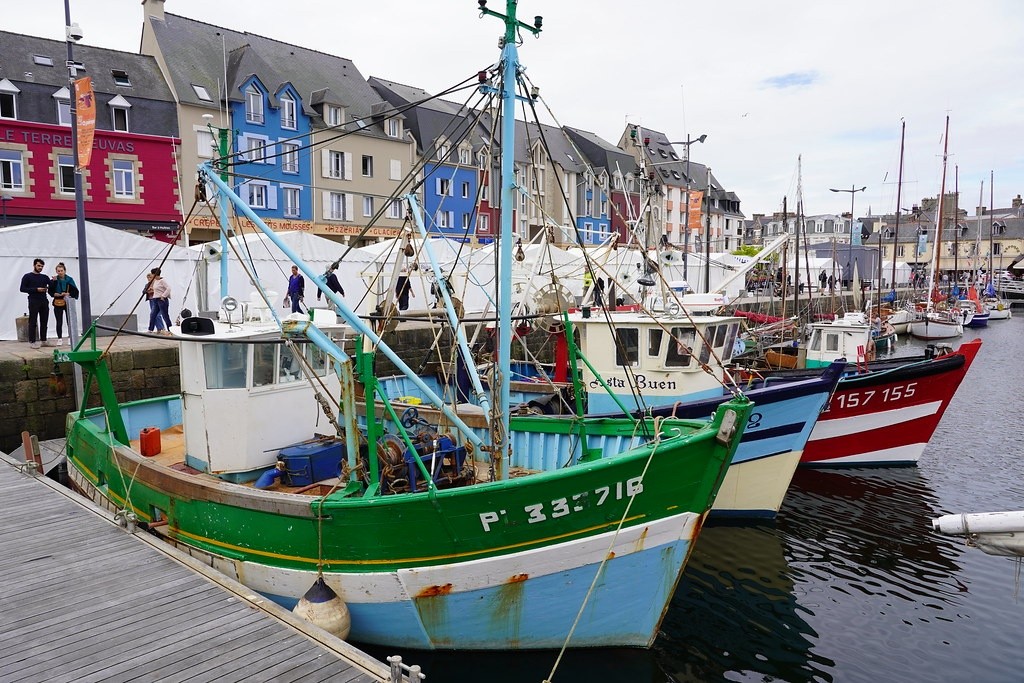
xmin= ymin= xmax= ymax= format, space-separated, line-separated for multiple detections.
xmin=39 ymin=340 xmax=54 ymax=346
xmin=30 ymin=342 xmax=39 ymax=348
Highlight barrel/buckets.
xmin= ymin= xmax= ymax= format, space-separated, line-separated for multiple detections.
xmin=140 ymin=427 xmax=161 ymax=457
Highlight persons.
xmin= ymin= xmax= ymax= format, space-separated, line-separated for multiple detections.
xmin=286 ymin=264 xmax=345 ymax=314
xmin=20 ymin=259 xmax=79 ymax=349
xmin=939 ymin=272 xmax=943 ymax=285
xmin=868 ymin=314 xmax=881 ymax=336
xmin=960 ymin=270 xmax=971 ymax=283
xmin=994 ymin=271 xmax=998 ymax=279
xmin=582 ymin=266 xmax=594 ymax=297
xmin=909 ymin=270 xmax=927 ymax=289
xmin=396 ymin=264 xmax=456 ymax=322
xmin=748 ymin=265 xmax=837 ymax=296
xmin=142 ymin=268 xmax=172 ymax=337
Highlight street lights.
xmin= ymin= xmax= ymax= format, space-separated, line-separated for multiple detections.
xmin=63 ymin=0 xmax=91 ymax=338
xmin=656 ymin=134 xmax=707 ymax=282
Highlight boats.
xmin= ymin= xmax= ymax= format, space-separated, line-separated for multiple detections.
xmin=479 ymin=182 xmax=983 ymax=466
xmin=53 ymin=1 xmax=754 ymax=647
xmin=363 ymin=192 xmax=846 ymax=522
xmin=728 ymin=115 xmax=1024 ymax=368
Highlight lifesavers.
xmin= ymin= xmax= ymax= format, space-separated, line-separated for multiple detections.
xmin=866 ymin=339 xmax=876 ymax=361
xmin=951 ymin=307 xmax=961 ymax=314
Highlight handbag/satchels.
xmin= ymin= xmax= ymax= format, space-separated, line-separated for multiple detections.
xmin=282 ymin=298 xmax=289 ymax=306
xmin=54 ymin=300 xmax=65 ymax=305
